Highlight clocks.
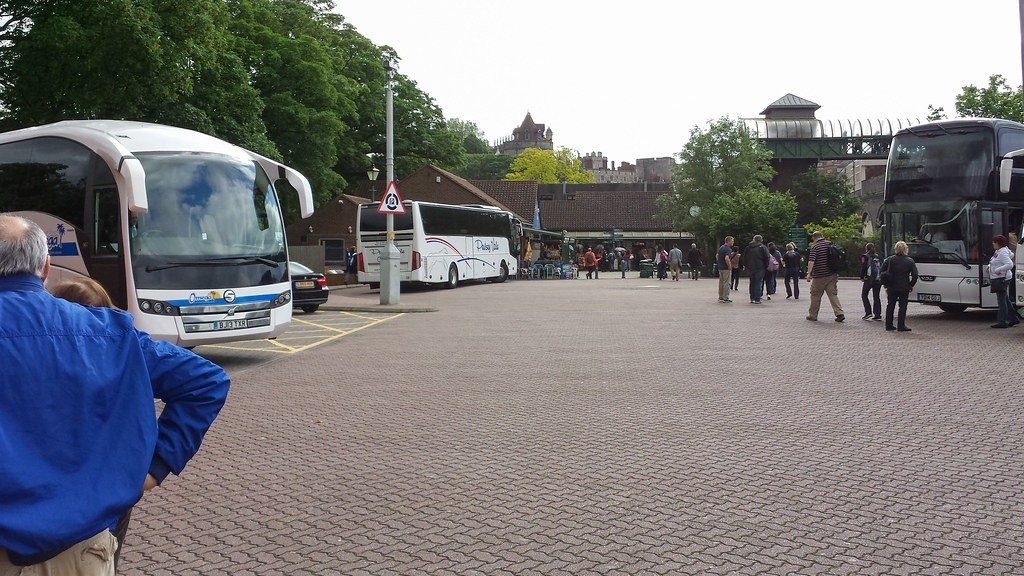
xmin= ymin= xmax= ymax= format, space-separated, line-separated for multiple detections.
xmin=689 ymin=204 xmax=702 ymax=217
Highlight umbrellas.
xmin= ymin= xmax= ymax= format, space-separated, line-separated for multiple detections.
xmin=524 ymin=242 xmax=532 ymax=280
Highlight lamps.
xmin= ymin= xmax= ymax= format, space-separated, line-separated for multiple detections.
xmin=309 ymin=225 xmax=313 ymax=233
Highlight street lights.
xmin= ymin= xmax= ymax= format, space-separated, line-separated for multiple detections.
xmin=365 ymin=163 xmax=381 ymax=202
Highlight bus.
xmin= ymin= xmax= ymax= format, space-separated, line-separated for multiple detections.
xmin=0 ymin=118 xmax=315 ymax=353
xmin=354 ymin=196 xmax=522 ymax=290
xmin=873 ymin=117 xmax=1024 ymax=321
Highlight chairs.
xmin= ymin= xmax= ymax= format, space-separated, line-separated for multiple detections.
xmin=515 ymin=264 xmax=561 ymax=280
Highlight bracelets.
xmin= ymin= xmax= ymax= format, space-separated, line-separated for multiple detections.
xmin=807 ymin=272 xmax=810 ymax=274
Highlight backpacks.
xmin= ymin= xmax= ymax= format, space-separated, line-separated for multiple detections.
xmin=766 ymin=254 xmax=779 ymax=272
xmin=864 ymin=253 xmax=881 ymax=281
xmin=586 ymin=253 xmax=594 ymax=264
xmin=828 ymin=241 xmax=846 ymax=273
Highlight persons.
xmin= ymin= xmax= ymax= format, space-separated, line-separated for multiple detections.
xmin=741 ymin=235 xmax=770 ymax=304
xmin=52 ymin=278 xmax=133 ymax=576
xmin=344 ymin=246 xmax=357 ymax=285
xmin=729 ymin=246 xmax=742 ymax=290
xmin=655 ymin=245 xmax=668 ymax=281
xmin=860 ymin=243 xmax=882 ymax=320
xmin=878 ymin=241 xmax=918 ymax=331
xmin=687 ymin=243 xmax=701 ymax=280
xmin=986 ymin=235 xmax=1020 ymax=329
xmin=924 ymin=226 xmax=947 ymax=242
xmin=806 ymin=232 xmax=846 ymax=322
xmin=761 ymin=242 xmax=804 ymax=300
xmin=601 ymin=247 xmax=653 ymax=272
xmin=669 ymin=244 xmax=682 ymax=281
xmin=716 ymin=237 xmax=734 ymax=302
xmin=1008 ymin=232 xmax=1018 ymax=301
xmin=0 ymin=215 xmax=230 ymax=576
xmin=574 ymin=243 xmax=583 ymax=256
xmin=584 ymin=248 xmax=596 ymax=279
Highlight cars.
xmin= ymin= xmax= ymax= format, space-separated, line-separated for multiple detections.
xmin=289 ymin=260 xmax=330 ymax=313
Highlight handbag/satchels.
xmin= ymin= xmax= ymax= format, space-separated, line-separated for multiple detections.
xmin=991 ymin=278 xmax=1005 ymax=293
xmin=678 ymin=261 xmax=683 ymax=267
xmin=661 ymin=250 xmax=668 ymax=262
xmin=880 ymin=259 xmax=890 ymax=285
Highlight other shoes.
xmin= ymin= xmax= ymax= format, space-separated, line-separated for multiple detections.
xmin=586 ymin=274 xmax=588 ymax=279
xmin=1008 ymin=318 xmax=1020 ymax=326
xmin=886 ymin=325 xmax=896 ymax=331
xmin=767 ymin=295 xmax=770 ymax=300
xmin=752 ymin=299 xmax=761 ymax=304
xmin=990 ymin=322 xmax=1008 ymax=328
xmin=806 ymin=315 xmax=817 ymax=321
xmin=872 ymin=316 xmax=882 ymax=320
xmin=718 ymin=297 xmax=733 ymax=303
xmin=787 ymin=294 xmax=792 ymax=298
xmin=795 ymin=296 xmax=798 ymax=299
xmin=862 ymin=314 xmax=872 ymax=320
xmin=835 ymin=314 xmax=844 ymax=322
xmin=898 ymin=327 xmax=911 ymax=331
